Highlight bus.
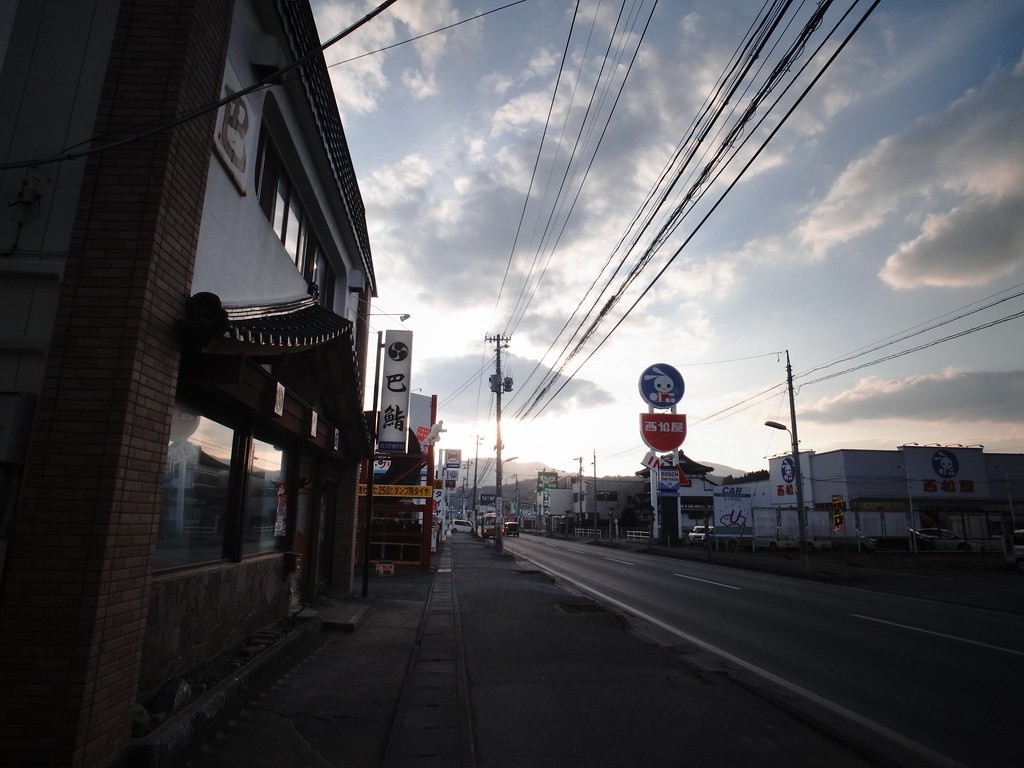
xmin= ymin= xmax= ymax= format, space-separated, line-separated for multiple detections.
xmin=481 ymin=513 xmax=504 ymax=539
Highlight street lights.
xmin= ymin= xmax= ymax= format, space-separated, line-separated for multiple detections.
xmin=994 ymin=465 xmax=1019 ymax=531
xmin=764 ymin=421 xmax=808 ymax=554
xmin=496 ymin=455 xmax=520 ymax=555
xmin=897 ymin=464 xmax=917 ymax=556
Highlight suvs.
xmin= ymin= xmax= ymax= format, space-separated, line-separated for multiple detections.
xmin=689 ymin=525 xmax=714 ymax=546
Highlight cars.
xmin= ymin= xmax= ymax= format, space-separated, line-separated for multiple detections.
xmin=431 ymin=517 xmax=451 ymax=539
xmin=1003 ymin=529 xmax=1024 ymax=573
xmin=910 ymin=527 xmax=978 ymax=551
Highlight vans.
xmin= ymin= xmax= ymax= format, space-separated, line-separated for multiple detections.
xmin=503 ymin=522 xmax=520 ymax=537
xmin=450 ymin=518 xmax=475 ymax=536
